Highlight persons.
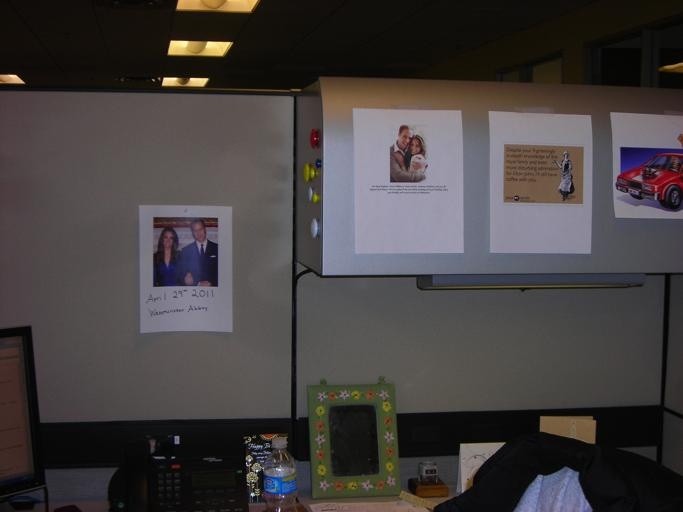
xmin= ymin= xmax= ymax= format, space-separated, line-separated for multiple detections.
xmin=390 ymin=125 xmax=429 ymax=181
xmin=153 ymin=219 xmax=218 ymax=286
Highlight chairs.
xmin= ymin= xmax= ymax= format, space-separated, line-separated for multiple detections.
xmin=437 ymin=431 xmax=682 ymax=512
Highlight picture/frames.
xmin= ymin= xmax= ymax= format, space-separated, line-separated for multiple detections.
xmin=0 ymin=325 xmax=46 ymax=498
xmin=305 ymin=384 xmax=401 ymax=500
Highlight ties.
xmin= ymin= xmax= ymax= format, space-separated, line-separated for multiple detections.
xmin=200 ymin=243 xmax=204 ymax=257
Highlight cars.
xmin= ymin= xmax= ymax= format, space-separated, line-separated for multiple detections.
xmin=613 ymin=146 xmax=683 ymax=214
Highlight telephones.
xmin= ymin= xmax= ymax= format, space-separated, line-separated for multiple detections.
xmin=118 ymin=434 xmax=250 ymax=512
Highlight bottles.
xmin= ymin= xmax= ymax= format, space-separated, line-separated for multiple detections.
xmin=265 ymin=437 xmax=299 ymax=510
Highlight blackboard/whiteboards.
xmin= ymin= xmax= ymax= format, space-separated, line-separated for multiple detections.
xmin=0 ymin=84 xmax=297 ymax=425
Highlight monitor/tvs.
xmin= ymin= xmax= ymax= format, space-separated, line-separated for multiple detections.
xmin=0 ymin=324 xmax=50 ymax=512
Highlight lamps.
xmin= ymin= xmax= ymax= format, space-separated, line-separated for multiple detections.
xmin=161 ymin=76 xmax=210 ymax=88
xmin=0 ymin=74 xmax=26 ymax=86
xmin=175 ymin=0 xmax=261 ymax=14
xmin=166 ymin=40 xmax=234 ymax=58
